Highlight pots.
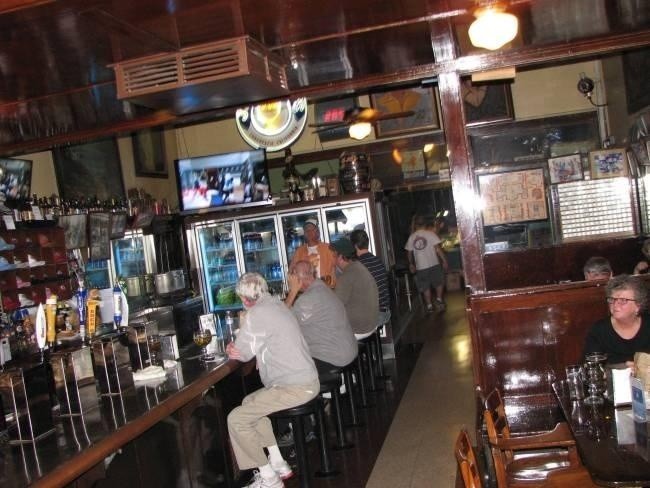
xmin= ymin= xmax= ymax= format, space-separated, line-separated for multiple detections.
xmin=155 ymin=268 xmax=192 ymax=294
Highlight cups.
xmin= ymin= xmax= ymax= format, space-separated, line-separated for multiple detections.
xmin=567 ymin=365 xmax=584 ymax=396
xmin=571 ymin=399 xmax=585 ymax=433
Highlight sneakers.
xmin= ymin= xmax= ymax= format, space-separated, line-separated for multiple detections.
xmin=434 ymin=297 xmax=447 ymax=308
xmin=241 ymin=470 xmax=285 ymax=488
xmin=276 ymin=434 xmax=295 ymax=447
xmin=272 ymin=460 xmax=294 ymax=480
xmin=427 ymin=306 xmax=434 ymax=313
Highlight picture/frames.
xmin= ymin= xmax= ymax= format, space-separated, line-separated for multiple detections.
xmin=547 ymin=146 xmax=630 ymax=187
xmin=367 ymin=77 xmax=519 ymax=140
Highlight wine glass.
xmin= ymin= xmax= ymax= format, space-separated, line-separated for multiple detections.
xmin=193 ymin=328 xmax=215 ymax=360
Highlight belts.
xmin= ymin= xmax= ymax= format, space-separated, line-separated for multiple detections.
xmin=379 ymin=305 xmax=392 ymax=312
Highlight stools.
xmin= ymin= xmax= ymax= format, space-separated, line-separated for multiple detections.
xmin=272 ymin=319 xmax=389 ymax=488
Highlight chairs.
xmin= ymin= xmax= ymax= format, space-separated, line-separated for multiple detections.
xmin=454 ymin=382 xmax=591 ymax=488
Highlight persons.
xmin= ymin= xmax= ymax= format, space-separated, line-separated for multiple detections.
xmin=179 ymin=167 xmax=270 ymax=210
xmin=274 ymin=260 xmax=358 ymax=448
xmin=223 ymin=271 xmax=320 ymax=488
xmin=404 ymin=216 xmax=450 ymax=314
xmin=578 ymin=254 xmax=612 ymax=284
xmin=316 ymin=238 xmax=382 ymax=399
xmin=289 ymin=220 xmax=342 ymax=289
xmin=624 ymin=234 xmax=650 ymax=277
xmin=346 ymin=229 xmax=393 ymax=326
xmin=580 ymin=274 xmax=650 ymax=369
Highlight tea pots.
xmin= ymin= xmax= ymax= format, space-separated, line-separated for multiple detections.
xmin=586 ymin=395 xmax=606 ymax=439
xmin=586 ymin=352 xmax=606 ymax=393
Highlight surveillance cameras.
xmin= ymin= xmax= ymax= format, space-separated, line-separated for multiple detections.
xmin=577 ymin=72 xmax=595 ymax=96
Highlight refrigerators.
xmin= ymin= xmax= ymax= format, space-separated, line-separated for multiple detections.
xmin=195 ymin=198 xmax=386 ymax=312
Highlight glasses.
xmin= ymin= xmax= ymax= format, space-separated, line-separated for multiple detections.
xmin=606 ymin=296 xmax=638 ymax=304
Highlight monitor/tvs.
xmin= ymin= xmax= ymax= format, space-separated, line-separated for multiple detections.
xmin=174 ymin=147 xmax=271 ymax=217
xmin=0 ymin=156 xmax=33 ymax=208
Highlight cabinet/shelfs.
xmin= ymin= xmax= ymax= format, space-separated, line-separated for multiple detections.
xmin=0 ymin=227 xmax=72 ymax=315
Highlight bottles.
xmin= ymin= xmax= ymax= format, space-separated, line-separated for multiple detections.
xmin=224 ymin=309 xmax=235 ymax=337
xmin=200 ymin=220 xmax=371 ymax=303
xmin=13 ymin=188 xmax=173 ymax=219
xmin=0 ymin=306 xmax=40 ymax=367
